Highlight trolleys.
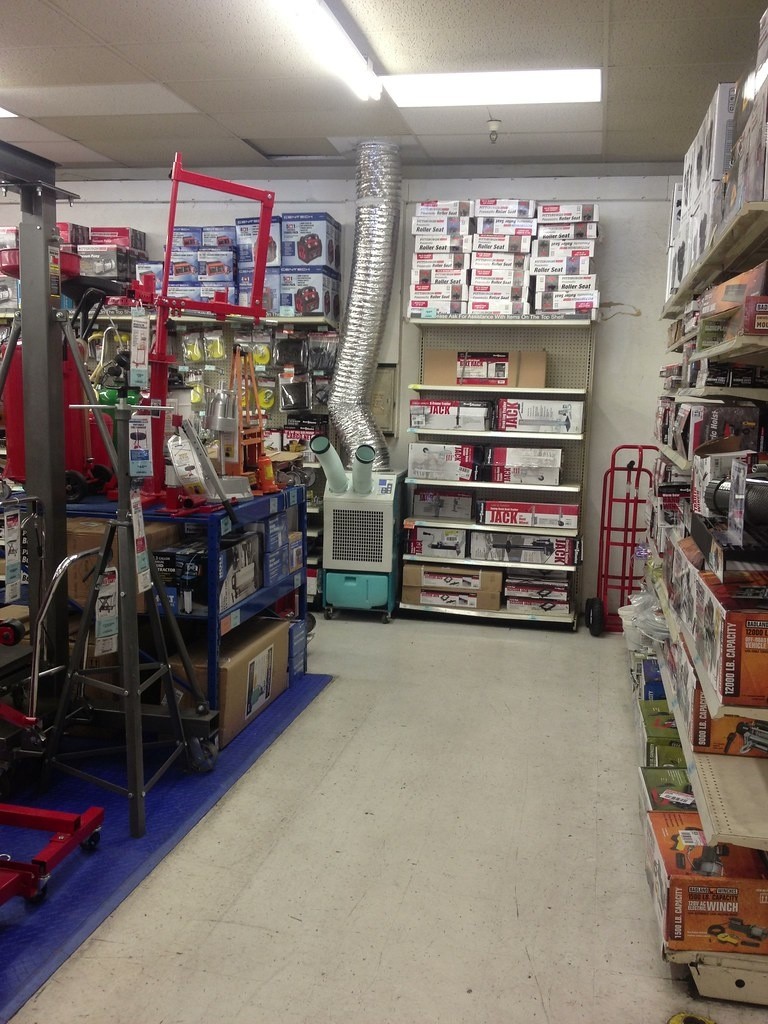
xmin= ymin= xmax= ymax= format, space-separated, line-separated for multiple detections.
xmin=585 ymin=444 xmax=660 ymax=637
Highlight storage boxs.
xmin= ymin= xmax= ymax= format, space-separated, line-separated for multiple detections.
xmin=634 ymin=657 xmax=768 ymax=957
xmin=1 ymin=211 xmax=346 ymax=322
xmin=661 ymin=13 xmax=768 ymax=302
xmin=63 ymin=622 xmax=122 ymax=703
xmin=674 ymin=632 xmax=767 ymax=762
xmin=290 ymin=617 xmax=307 ymax=657
xmin=261 ymin=543 xmax=290 ymax=589
xmin=156 ymin=618 xmax=290 ymax=746
xmin=406 ymin=398 xmax=585 ymax=614
xmin=233 ymin=510 xmax=290 ymax=553
xmin=698 ymin=258 xmax=767 ymax=321
xmin=291 ymin=649 xmax=305 ymax=689
xmin=150 ymin=530 xmax=264 ymax=621
xmin=60 ymin=514 xmax=177 ymax=612
xmin=688 ymin=573 xmax=768 ymax=709
xmin=288 ymin=531 xmax=305 ymax=574
xmin=406 ymin=198 xmax=604 ymax=323
xmin=720 ymin=295 xmax=768 ymax=342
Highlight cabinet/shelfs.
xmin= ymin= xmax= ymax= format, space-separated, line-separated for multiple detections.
xmin=624 ymin=201 xmax=768 ymax=975
xmin=0 ymin=307 xmax=352 ymax=745
xmin=395 ymin=316 xmax=593 ymax=629
